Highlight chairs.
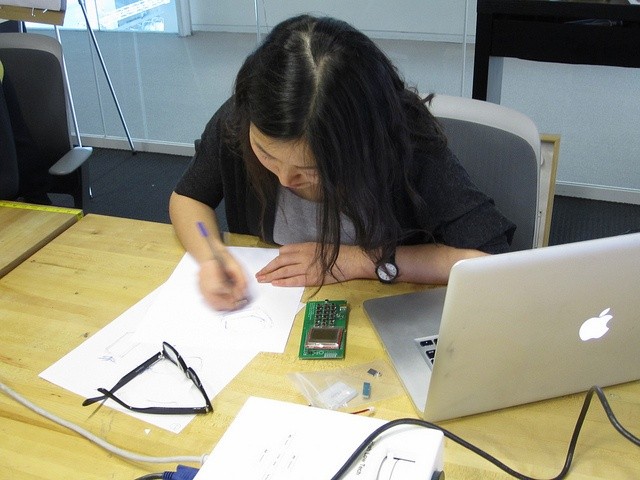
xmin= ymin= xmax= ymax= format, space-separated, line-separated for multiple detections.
xmin=420 ymin=87 xmax=542 ymax=254
xmin=0 ymin=32 xmax=92 ymax=207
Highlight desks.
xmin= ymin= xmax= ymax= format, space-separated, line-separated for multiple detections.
xmin=0 ymin=199 xmax=84 ymax=277
xmin=0 ymin=214 xmax=640 ymax=480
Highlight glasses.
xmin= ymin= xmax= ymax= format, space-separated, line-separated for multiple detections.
xmin=82 ymin=342 xmax=215 ymax=414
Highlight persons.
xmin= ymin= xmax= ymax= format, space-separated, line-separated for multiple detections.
xmin=167 ymin=15 xmax=516 ymax=312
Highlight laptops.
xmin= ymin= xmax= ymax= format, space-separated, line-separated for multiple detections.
xmin=361 ymin=231 xmax=639 ymax=425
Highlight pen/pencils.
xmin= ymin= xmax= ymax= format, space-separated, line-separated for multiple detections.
xmin=197 ymin=222 xmax=250 ymax=303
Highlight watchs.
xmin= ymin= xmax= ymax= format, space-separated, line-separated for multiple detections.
xmin=375 ymin=244 xmax=399 ymax=284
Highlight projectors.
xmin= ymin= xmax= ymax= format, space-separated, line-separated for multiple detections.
xmin=190 ymin=394 xmax=447 ymax=480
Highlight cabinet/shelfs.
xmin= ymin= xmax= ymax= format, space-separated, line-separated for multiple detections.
xmin=184 ymin=0 xmax=477 ymax=46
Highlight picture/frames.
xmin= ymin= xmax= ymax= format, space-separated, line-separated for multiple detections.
xmin=535 ymin=133 xmax=563 ymax=247
